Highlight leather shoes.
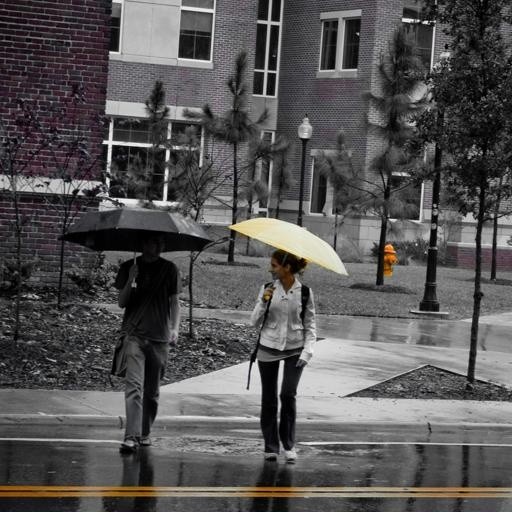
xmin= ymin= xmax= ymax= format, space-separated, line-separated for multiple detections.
xmin=139 ymin=437 xmax=151 ymax=446
xmin=120 ymin=438 xmax=139 ymax=448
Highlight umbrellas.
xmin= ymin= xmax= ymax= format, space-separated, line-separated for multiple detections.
xmin=57 ymin=208 xmax=214 ymax=288
xmin=227 ymin=217 xmax=349 ymax=302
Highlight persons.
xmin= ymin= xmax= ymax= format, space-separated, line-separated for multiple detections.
xmin=250 ymin=461 xmax=293 ymax=512
xmin=250 ymin=249 xmax=318 ymax=458
xmin=114 ymin=232 xmax=185 ymax=450
xmin=113 ymin=447 xmax=156 ymax=512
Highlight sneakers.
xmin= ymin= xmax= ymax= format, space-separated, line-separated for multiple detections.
xmin=283 ymin=447 xmax=297 ymax=461
xmin=263 ymin=451 xmax=277 ymax=460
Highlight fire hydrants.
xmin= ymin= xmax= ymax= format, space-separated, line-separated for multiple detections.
xmin=382 ymin=243 xmax=397 ymax=277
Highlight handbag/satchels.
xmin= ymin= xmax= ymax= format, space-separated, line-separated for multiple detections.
xmin=110 ymin=331 xmax=130 ymax=377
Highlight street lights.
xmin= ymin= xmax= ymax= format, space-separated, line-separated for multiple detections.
xmin=297 ymin=115 xmax=313 ymax=227
xmin=419 ymin=44 xmax=453 ymax=317
